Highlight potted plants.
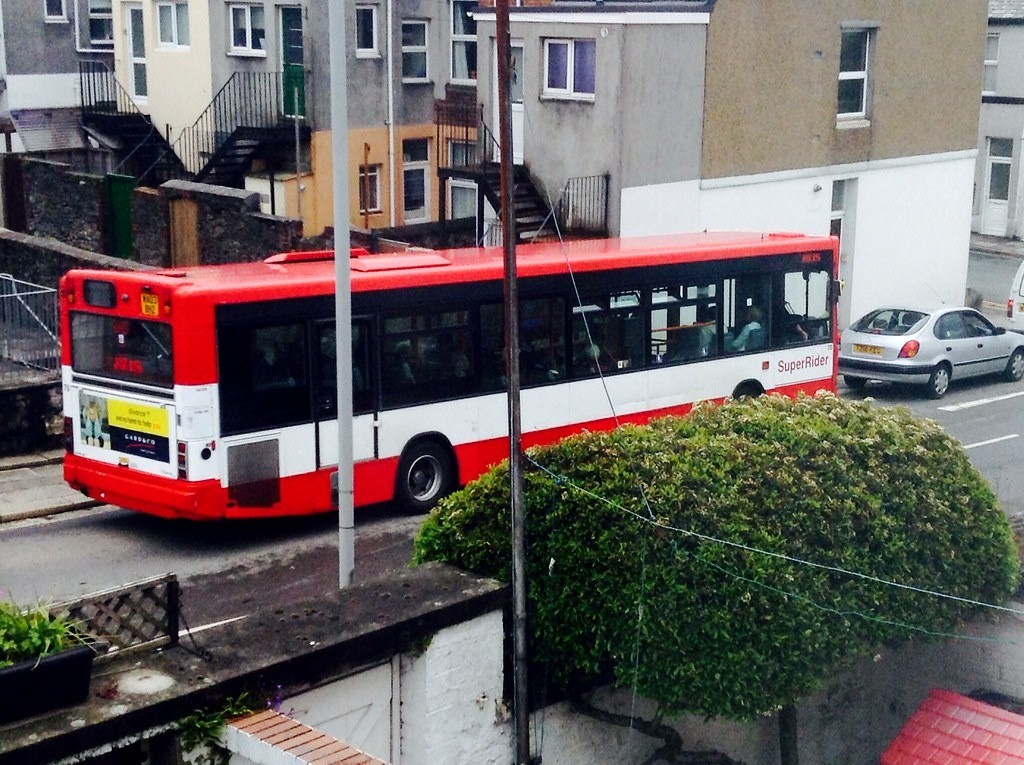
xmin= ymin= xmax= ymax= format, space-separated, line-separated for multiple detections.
xmin=0 ymin=590 xmax=117 ymax=728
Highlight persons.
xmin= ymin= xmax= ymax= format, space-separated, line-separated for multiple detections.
xmin=396 ymin=345 xmax=607 ymax=385
xmin=658 ymin=305 xmax=765 ymax=363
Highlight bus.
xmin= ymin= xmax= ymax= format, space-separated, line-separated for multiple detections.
xmin=58 ymin=232 xmax=841 ymax=522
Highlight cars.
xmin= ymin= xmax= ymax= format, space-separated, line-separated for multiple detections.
xmin=838 ymin=304 xmax=1024 ymax=400
